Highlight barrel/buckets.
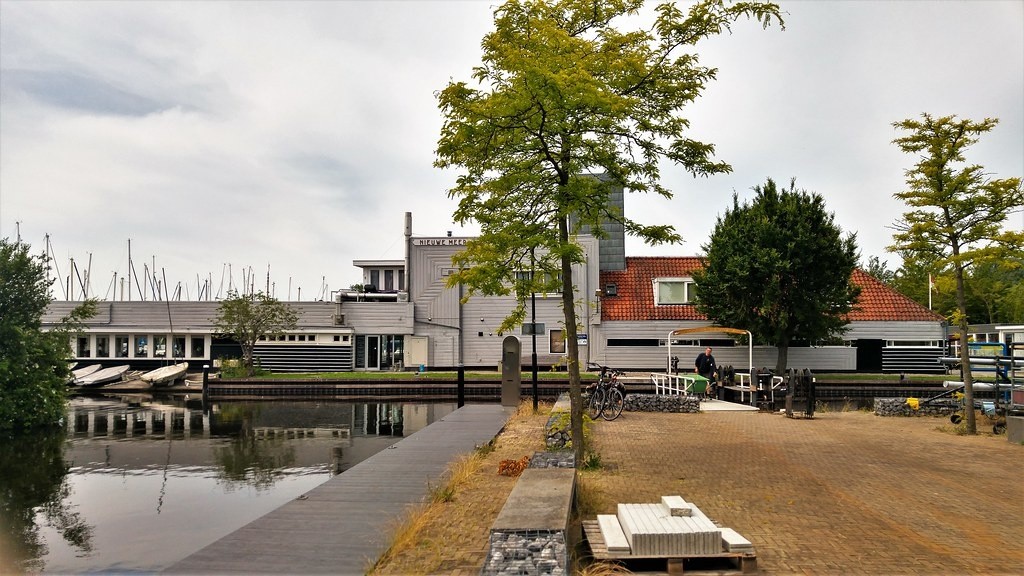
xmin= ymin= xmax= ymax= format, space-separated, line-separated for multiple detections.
xmin=906 ymin=398 xmax=918 ymax=410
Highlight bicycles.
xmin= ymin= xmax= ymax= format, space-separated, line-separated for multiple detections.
xmin=601 ymin=370 xmax=626 ymax=422
xmin=586 ymin=362 xmax=617 ymax=420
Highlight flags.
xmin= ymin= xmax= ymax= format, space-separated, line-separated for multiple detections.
xmin=931 ymin=277 xmax=938 ymax=294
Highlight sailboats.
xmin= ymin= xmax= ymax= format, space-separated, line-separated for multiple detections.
xmin=139 ymin=268 xmax=189 ymax=386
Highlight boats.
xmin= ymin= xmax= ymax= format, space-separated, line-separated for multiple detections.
xmin=73 ymin=365 xmax=131 ymax=386
xmin=68 ymin=363 xmax=78 ymax=370
xmin=64 ymin=363 xmax=102 ymax=385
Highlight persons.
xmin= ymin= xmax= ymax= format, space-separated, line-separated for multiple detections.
xmin=695 ymin=347 xmax=717 ymax=400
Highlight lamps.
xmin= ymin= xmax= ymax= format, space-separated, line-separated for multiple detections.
xmin=595 ymin=288 xmax=601 ymax=296
